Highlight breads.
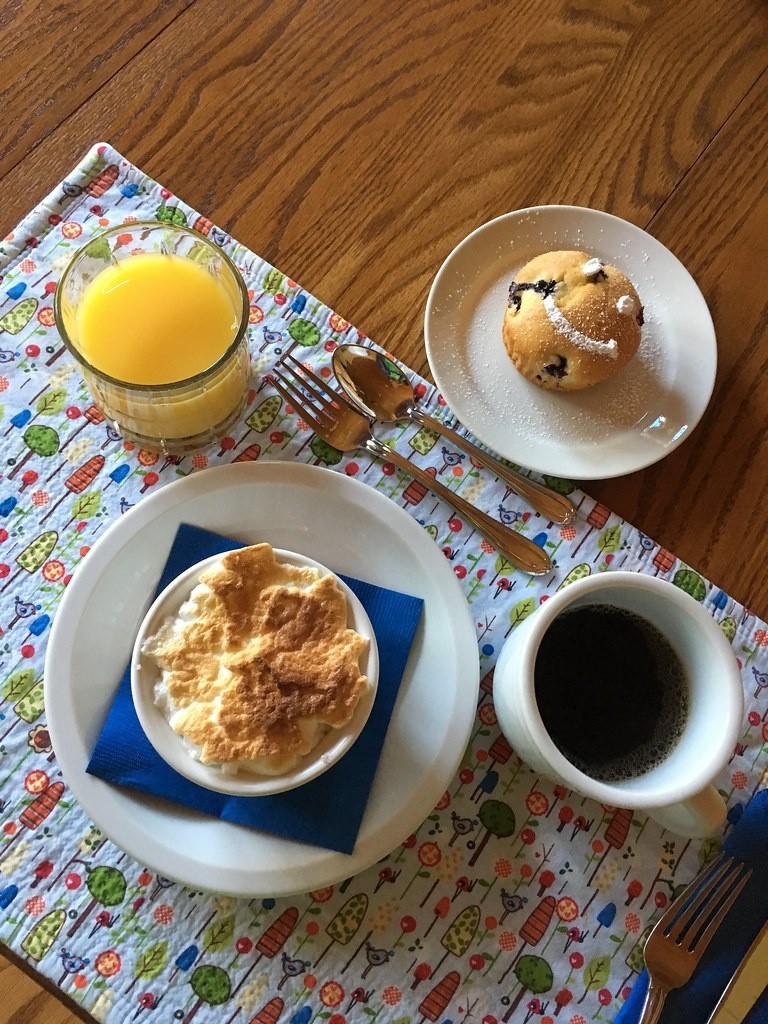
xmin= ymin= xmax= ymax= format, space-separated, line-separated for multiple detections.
xmin=503 ymin=250 xmax=644 ymax=392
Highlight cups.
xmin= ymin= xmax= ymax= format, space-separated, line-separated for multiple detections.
xmin=492 ymin=573 xmax=744 ymax=840
xmin=55 ymin=221 xmax=249 ymax=457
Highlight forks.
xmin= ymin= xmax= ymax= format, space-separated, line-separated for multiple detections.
xmin=266 ymin=355 xmax=551 ymax=576
xmin=636 ymin=850 xmax=752 ymax=1024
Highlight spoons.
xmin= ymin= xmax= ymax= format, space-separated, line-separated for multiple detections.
xmin=331 ymin=345 xmax=578 ymax=525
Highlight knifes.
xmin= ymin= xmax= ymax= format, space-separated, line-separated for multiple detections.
xmin=707 ymin=919 xmax=768 ymax=1024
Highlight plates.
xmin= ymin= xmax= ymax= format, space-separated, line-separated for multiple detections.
xmin=44 ymin=461 xmax=480 ymax=897
xmin=424 ymin=204 xmax=717 ymax=479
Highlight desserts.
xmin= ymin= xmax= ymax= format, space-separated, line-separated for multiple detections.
xmin=140 ymin=542 xmax=371 ymax=777
